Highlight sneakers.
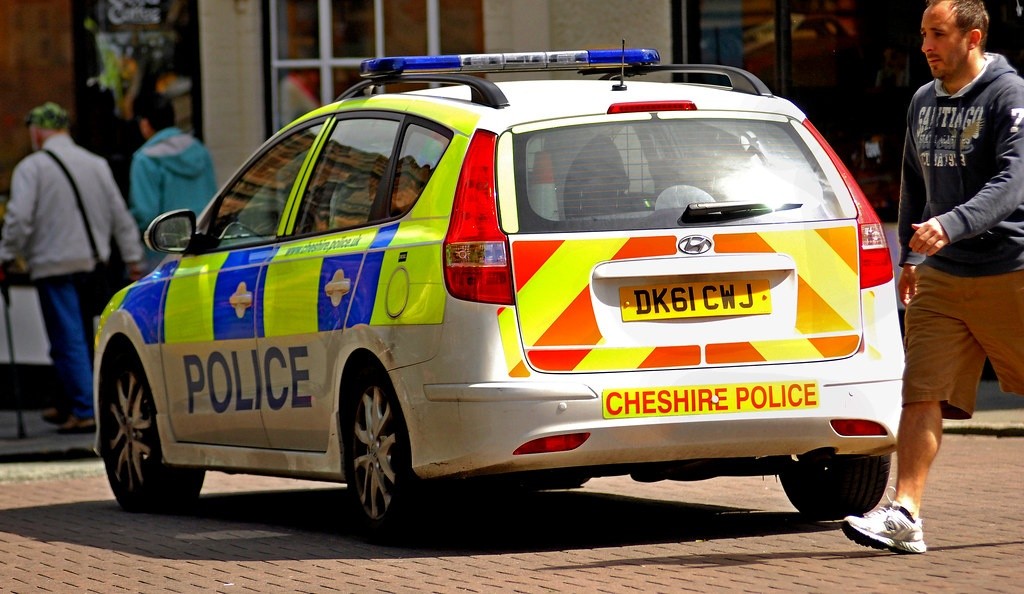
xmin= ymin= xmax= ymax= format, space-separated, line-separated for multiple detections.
xmin=841 ymin=487 xmax=926 ymax=553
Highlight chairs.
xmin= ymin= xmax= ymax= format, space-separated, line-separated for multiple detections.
xmin=675 ymin=123 xmax=755 ymax=205
xmin=545 ymin=132 xmax=630 ymax=218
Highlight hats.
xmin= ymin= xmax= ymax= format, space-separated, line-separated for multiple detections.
xmin=25 ymin=103 xmax=71 ymax=130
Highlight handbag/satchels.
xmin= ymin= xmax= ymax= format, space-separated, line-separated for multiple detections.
xmin=83 ymin=259 xmax=132 ymax=315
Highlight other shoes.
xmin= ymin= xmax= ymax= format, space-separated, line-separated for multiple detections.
xmin=58 ymin=419 xmax=95 ymax=434
xmin=41 ymin=405 xmax=69 ymax=422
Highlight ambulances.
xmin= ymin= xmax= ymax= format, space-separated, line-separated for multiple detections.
xmin=90 ymin=49 xmax=909 ymax=547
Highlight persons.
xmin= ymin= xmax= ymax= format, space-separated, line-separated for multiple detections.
xmin=0 ymin=99 xmax=143 ymax=434
xmin=842 ymin=0 xmax=1024 ymax=556
xmin=127 ymin=92 xmax=218 ymax=272
